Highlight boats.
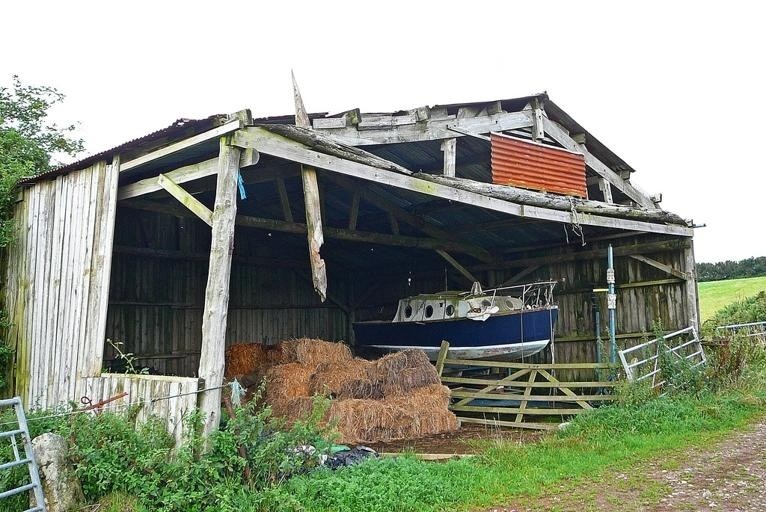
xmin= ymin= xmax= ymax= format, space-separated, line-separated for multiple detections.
xmin=351 ymin=277 xmax=560 ymax=362
